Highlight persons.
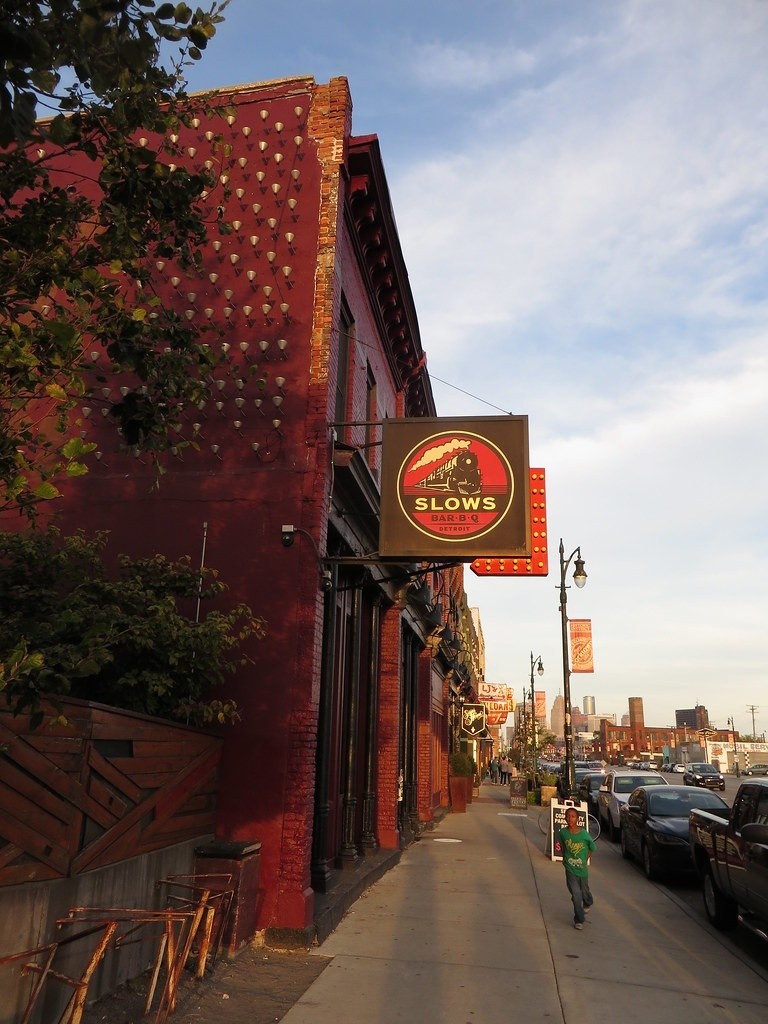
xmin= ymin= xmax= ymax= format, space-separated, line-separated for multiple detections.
xmin=490 ymin=757 xmax=512 ymax=786
xmin=559 ymin=808 xmax=596 ymax=930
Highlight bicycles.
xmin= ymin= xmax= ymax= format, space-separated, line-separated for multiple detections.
xmin=539 ymin=804 xmax=602 ymax=843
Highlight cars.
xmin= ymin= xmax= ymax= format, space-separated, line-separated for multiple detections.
xmin=620 ymin=783 xmax=732 ymax=880
xmin=535 ymin=757 xmax=605 ymax=798
xmin=578 ymin=774 xmax=604 ymax=810
xmin=626 ymin=761 xmax=684 ymax=774
xmin=743 ymin=763 xmax=768 ymax=776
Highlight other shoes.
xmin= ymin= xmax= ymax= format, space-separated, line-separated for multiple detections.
xmin=575 ymin=922 xmax=583 ymax=930
xmin=504 ymin=784 xmax=506 ymax=786
xmin=495 ymin=783 xmax=498 ymax=785
xmin=500 ymin=784 xmax=502 ymax=786
xmin=492 ymin=782 xmax=495 ymax=784
xmin=583 ymin=902 xmax=590 ymax=913
xmin=509 ymin=784 xmax=510 ymax=786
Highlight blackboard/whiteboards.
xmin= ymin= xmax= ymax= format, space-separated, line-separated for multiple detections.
xmin=544 ymin=798 xmax=590 ymax=866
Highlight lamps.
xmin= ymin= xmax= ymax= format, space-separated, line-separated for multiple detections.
xmin=406 ymin=573 xmax=471 ymax=696
xmin=36 ymin=149 xmax=51 ymax=171
xmin=41 ymin=306 xmax=55 ymax=320
xmin=81 ymin=107 xmax=304 ymax=466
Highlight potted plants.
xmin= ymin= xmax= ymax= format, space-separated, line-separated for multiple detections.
xmin=448 ymin=752 xmax=481 ymax=813
xmin=540 ymin=773 xmax=557 ymax=807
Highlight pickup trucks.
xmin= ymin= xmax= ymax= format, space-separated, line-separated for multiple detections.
xmin=688 ymin=779 xmax=768 ymax=943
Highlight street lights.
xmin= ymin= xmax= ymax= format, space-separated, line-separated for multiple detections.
xmin=630 ymin=735 xmax=638 ymax=758
xmin=515 ymin=711 xmax=523 ymax=772
xmin=523 ymin=689 xmax=532 ymax=769
xmin=555 ymin=544 xmax=587 ymax=797
xmin=728 ymin=718 xmax=740 ymax=778
xmin=531 ymin=655 xmax=545 ymax=767
xmin=671 ymin=726 xmax=678 ymax=761
xmin=646 ymin=733 xmax=653 ymax=760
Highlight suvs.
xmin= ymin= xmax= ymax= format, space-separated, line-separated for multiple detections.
xmin=683 ymin=762 xmax=725 ymax=791
xmin=645 ymin=762 xmax=658 ymax=771
xmin=597 ymin=770 xmax=671 ymax=840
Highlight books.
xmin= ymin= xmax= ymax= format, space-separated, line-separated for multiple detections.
xmin=195 ymin=840 xmax=261 ymax=855
